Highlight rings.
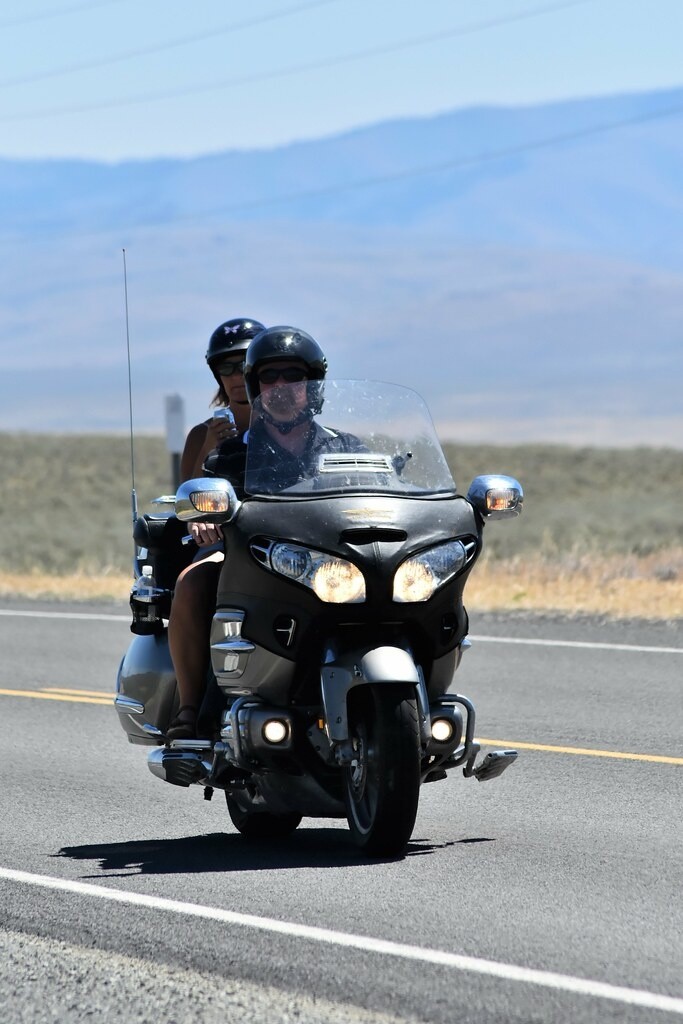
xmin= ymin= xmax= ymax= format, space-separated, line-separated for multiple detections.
xmin=220 ymin=433 xmax=222 ymax=438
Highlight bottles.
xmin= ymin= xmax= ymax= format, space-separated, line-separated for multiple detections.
xmin=137 ymin=565 xmax=156 ymax=622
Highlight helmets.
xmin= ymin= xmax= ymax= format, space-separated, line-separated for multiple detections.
xmin=205 ymin=318 xmax=266 ymax=374
xmin=242 ymin=325 xmax=328 ymax=410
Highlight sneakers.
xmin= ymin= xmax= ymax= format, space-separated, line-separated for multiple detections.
xmin=165 ymin=720 xmax=198 ymax=748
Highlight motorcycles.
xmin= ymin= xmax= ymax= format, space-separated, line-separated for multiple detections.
xmin=114 ymin=249 xmax=524 ymax=860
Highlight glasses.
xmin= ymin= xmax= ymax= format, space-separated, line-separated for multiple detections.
xmin=257 ymin=366 xmax=308 ymax=384
xmin=218 ymin=359 xmax=245 ymax=376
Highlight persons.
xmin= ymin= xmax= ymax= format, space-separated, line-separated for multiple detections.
xmin=164 ymin=319 xmax=469 ymax=784
